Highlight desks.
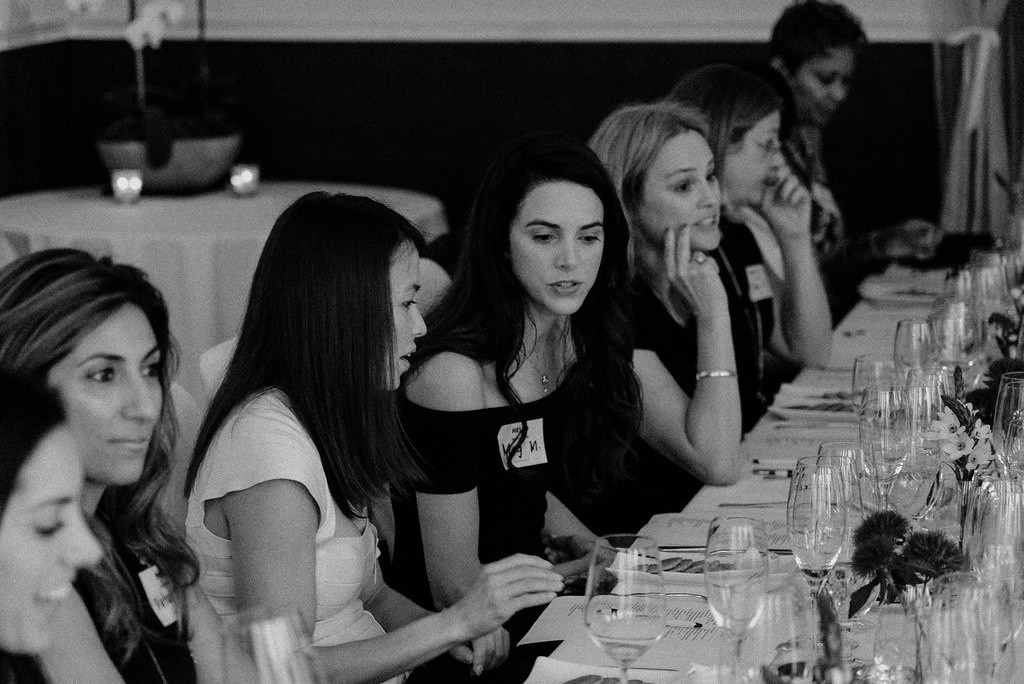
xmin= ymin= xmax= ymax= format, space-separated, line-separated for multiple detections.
xmin=0 ymin=178 xmax=447 ymax=389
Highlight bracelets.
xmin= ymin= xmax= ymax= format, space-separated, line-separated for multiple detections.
xmin=694 ymin=370 xmax=740 ymax=383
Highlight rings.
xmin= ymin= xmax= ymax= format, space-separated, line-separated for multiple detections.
xmin=689 ymin=256 xmax=704 ymax=264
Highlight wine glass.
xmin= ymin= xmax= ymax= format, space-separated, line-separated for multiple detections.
xmin=584 ymin=250 xmax=1024 ymax=684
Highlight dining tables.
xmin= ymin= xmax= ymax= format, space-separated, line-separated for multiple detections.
xmin=525 ymin=220 xmax=1024 ymax=684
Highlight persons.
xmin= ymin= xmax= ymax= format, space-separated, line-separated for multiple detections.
xmin=181 ymin=189 xmax=561 ymax=684
xmin=768 ymin=1 xmax=945 ymax=324
xmin=385 ymin=135 xmax=649 ymax=684
xmin=551 ymin=104 xmax=746 ymax=530
xmin=0 ymin=246 xmax=272 ymax=684
xmin=665 ymin=65 xmax=835 ymax=428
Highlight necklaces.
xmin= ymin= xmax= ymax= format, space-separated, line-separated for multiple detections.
xmin=519 ymin=335 xmax=555 ymax=394
xmin=713 ymin=240 xmax=767 ymax=405
xmin=3 ymin=371 xmax=107 ymax=683
